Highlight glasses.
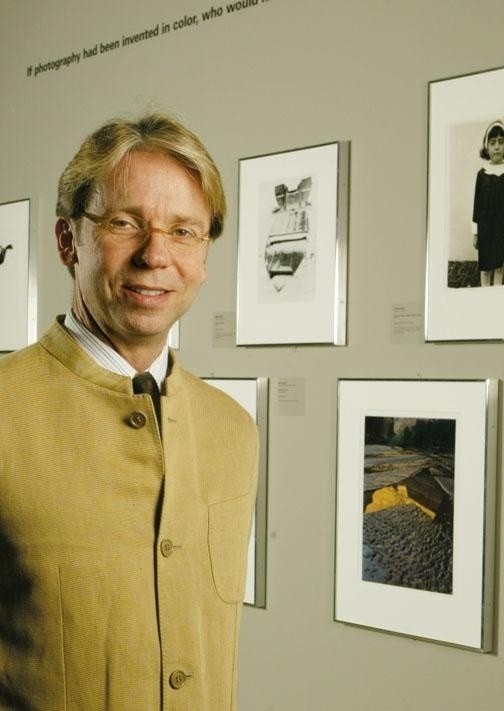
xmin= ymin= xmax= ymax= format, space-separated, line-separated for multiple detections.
xmin=79 ymin=207 xmax=210 ymax=245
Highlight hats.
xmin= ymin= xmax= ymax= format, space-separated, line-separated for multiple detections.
xmin=480 ymin=118 xmax=504 ymax=150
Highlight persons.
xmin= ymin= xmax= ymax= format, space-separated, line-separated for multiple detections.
xmin=0 ymin=111 xmax=259 ymax=711
xmin=469 ymin=119 xmax=504 ymax=287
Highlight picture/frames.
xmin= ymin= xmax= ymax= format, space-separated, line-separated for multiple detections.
xmin=200 ymin=377 xmax=268 ymax=609
xmin=332 ymin=376 xmax=498 ymax=655
xmin=236 ymin=139 xmax=349 ymax=345
xmin=0 ymin=196 xmax=40 ymax=354
xmin=425 ymin=67 xmax=503 ymax=339
